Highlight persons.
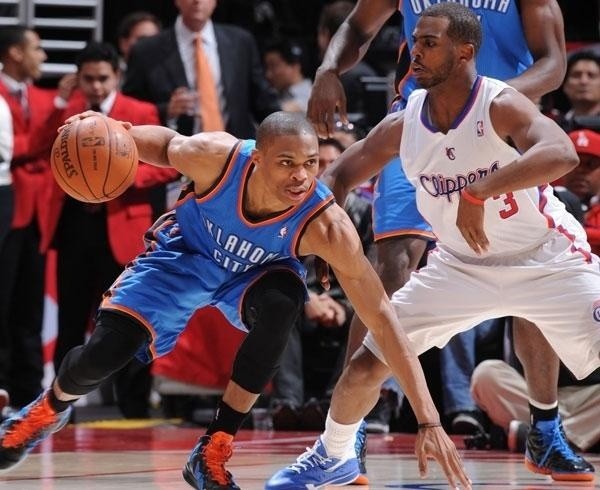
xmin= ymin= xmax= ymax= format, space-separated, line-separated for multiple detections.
xmin=123 ymin=0 xmax=280 ymax=422
xmin=267 ymin=12 xmax=504 ymax=435
xmin=464 ymin=186 xmax=600 ymax=460
xmin=1 ymin=15 xmax=189 ymax=422
xmin=265 ymin=4 xmax=599 ymax=489
xmin=306 ymin=1 xmax=567 ymax=472
xmin=1 ymin=110 xmax=473 ymax=490
xmin=543 ymin=46 xmax=598 ymax=253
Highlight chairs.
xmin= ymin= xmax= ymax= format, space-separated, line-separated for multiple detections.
xmin=359 ymin=76 xmax=387 ymax=127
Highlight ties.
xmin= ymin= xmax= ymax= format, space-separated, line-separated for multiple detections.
xmin=193 ymin=33 xmax=224 ymax=132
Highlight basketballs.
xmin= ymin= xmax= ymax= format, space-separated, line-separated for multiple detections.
xmin=51 ymin=116 xmax=137 ymax=204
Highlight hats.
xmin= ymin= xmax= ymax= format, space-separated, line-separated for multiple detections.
xmin=552 ymin=185 xmax=586 ymax=228
xmin=567 ymin=128 xmax=600 ymax=157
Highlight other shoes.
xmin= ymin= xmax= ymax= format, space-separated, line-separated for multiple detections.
xmin=508 ymin=420 xmax=530 ymax=452
xmin=362 ymin=396 xmax=390 ymax=432
xmin=302 ymin=398 xmax=325 ymax=431
xmin=272 ymin=401 xmax=299 ymax=431
xmin=453 ymin=412 xmax=493 ymax=448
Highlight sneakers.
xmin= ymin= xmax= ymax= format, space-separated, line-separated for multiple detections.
xmin=0 ymin=388 xmax=71 ymax=472
xmin=525 ymin=415 xmax=596 ymax=480
xmin=183 ymin=431 xmax=241 ymax=490
xmin=354 ymin=421 xmax=369 ymax=484
xmin=264 ymin=434 xmax=359 ymax=490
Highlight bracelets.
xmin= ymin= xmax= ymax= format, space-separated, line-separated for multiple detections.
xmin=461 ymin=187 xmax=484 ymax=206
